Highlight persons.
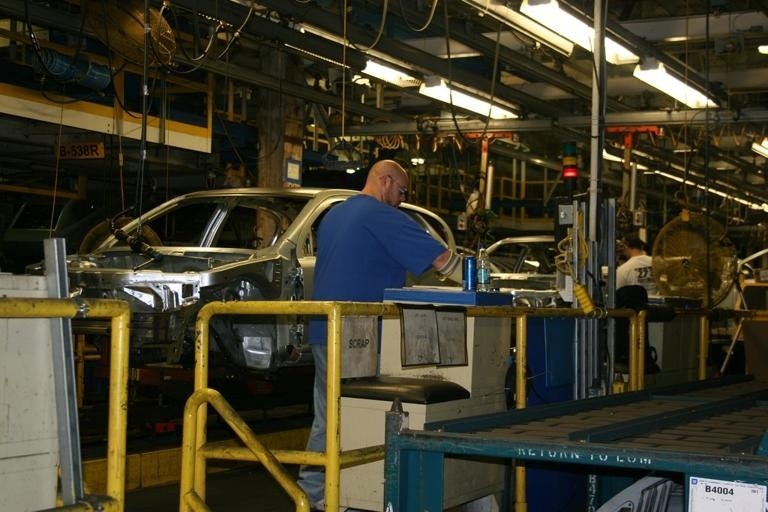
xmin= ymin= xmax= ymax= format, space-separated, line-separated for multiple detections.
xmin=616 ymin=235 xmax=667 ymax=298
xmin=295 ymin=158 xmax=464 ymax=511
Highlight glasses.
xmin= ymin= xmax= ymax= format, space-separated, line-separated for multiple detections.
xmin=379 ymin=173 xmax=408 ymax=196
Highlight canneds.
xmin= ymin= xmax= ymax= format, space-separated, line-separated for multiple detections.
xmin=462 ymin=255 xmax=477 ymax=292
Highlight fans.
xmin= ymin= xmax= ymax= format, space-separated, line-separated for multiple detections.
xmin=650 ymin=214 xmax=738 ymax=310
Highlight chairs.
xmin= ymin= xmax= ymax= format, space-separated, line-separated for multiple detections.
xmin=338 ymin=288 xmax=512 ymax=512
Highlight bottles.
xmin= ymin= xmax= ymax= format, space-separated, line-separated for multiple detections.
xmin=476 ymin=248 xmax=491 ymax=293
